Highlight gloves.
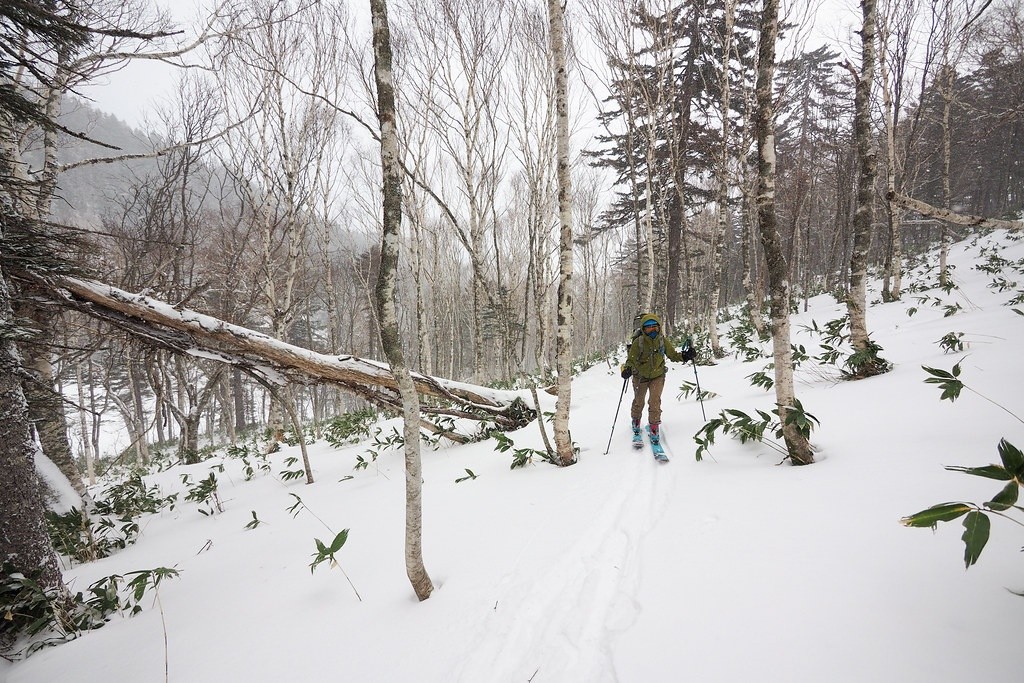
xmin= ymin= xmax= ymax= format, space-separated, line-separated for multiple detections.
xmin=682 ymin=347 xmax=697 ymax=362
xmin=621 ymin=366 xmax=632 ymax=379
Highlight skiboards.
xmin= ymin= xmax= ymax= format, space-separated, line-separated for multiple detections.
xmin=630 ymin=418 xmax=670 ymax=463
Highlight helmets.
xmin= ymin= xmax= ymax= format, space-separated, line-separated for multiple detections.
xmin=644 ymin=319 xmax=661 ymax=339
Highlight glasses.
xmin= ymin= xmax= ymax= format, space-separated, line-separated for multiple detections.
xmin=643 ymin=324 xmax=660 ymax=335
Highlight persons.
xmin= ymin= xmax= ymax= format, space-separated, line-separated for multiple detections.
xmin=620 ymin=314 xmax=696 ymax=444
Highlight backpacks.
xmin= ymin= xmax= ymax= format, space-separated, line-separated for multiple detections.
xmin=631 ymin=313 xmax=665 ymax=361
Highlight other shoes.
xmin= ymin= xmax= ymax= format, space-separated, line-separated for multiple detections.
xmin=632 ymin=419 xmax=641 ymax=433
xmin=649 ymin=424 xmax=660 ymax=443
